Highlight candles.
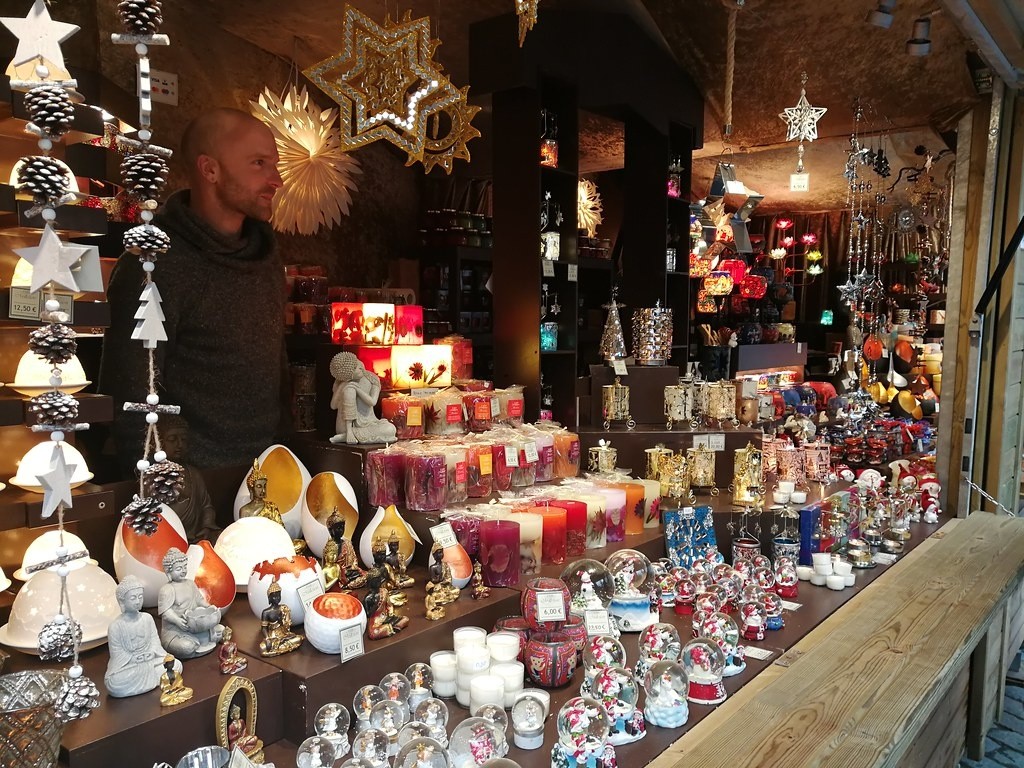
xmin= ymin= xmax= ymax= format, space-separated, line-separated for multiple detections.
xmin=330 ymin=301 xmax=663 ymax=586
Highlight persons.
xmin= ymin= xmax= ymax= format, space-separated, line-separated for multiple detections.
xmin=94 ymin=108 xmax=289 ymax=529
xmin=331 ymin=352 xmax=397 ymax=445
xmin=104 ymin=459 xmax=489 ymax=708
xmin=135 ymin=417 xmax=223 ymax=543
xmin=227 ymin=705 xmax=259 ymax=757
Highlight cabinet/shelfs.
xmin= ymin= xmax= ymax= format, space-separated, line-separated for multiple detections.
xmin=287 ymin=432 xmax=602 ymax=577
xmin=312 ymin=342 xmax=467 ymax=441
xmin=419 ymin=68 xmax=858 ymax=566
xmin=0 ymin=90 xmax=116 ymax=626
xmin=0 ymin=508 xmax=1024 ymax=768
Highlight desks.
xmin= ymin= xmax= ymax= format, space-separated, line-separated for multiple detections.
xmin=694 ymin=342 xmax=807 ymax=385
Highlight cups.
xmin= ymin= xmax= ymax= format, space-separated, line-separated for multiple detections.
xmin=797 ymin=553 xmax=857 ymax=589
xmin=772 ymin=537 xmax=800 ymax=568
xmin=731 ymin=537 xmax=761 ymax=566
xmin=773 ymin=481 xmax=806 ymax=504
xmin=368 ymin=390 xmax=662 ymax=585
xmin=429 ymin=628 xmax=551 ymax=721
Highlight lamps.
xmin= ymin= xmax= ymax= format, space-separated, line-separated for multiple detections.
xmin=865 ymin=0 xmax=896 ymax=30
xmin=690 ymin=214 xmax=822 ymax=319
xmin=904 ymin=7 xmax=944 ymax=56
